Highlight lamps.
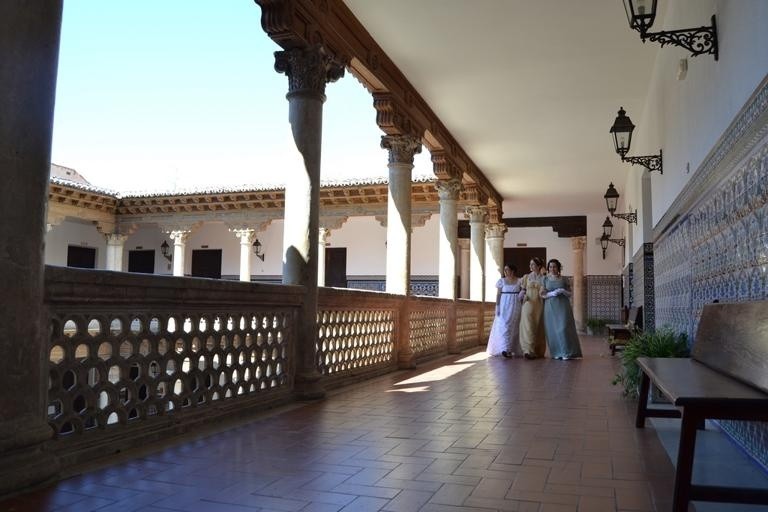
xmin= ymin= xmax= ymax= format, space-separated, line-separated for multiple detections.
xmin=252 ymin=238 xmax=265 ymax=262
xmin=599 ymin=232 xmax=608 ymax=260
xmin=600 ymin=215 xmax=625 ymax=249
xmin=160 ymin=239 xmax=172 ymax=262
xmin=607 ymin=106 xmax=663 ymax=176
xmin=621 ymin=0 xmax=719 ymax=63
xmin=602 ymin=182 xmax=637 ymax=226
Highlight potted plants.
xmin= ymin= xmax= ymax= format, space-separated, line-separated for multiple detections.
xmin=609 ymin=326 xmax=691 ymax=404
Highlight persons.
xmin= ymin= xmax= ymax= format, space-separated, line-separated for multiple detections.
xmin=516 ymin=255 xmax=547 ymax=359
xmin=486 ymin=262 xmax=526 ymax=359
xmin=539 ymin=259 xmax=584 ymax=361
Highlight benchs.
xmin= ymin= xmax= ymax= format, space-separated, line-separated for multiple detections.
xmin=633 ymin=298 xmax=767 ymax=511
xmin=604 ymin=305 xmax=642 ymax=356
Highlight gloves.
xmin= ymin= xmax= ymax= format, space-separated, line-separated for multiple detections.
xmin=496 ymin=305 xmax=500 ymax=316
xmin=518 ymin=290 xmax=526 ymax=302
xmin=547 ymin=288 xmax=571 ymax=298
